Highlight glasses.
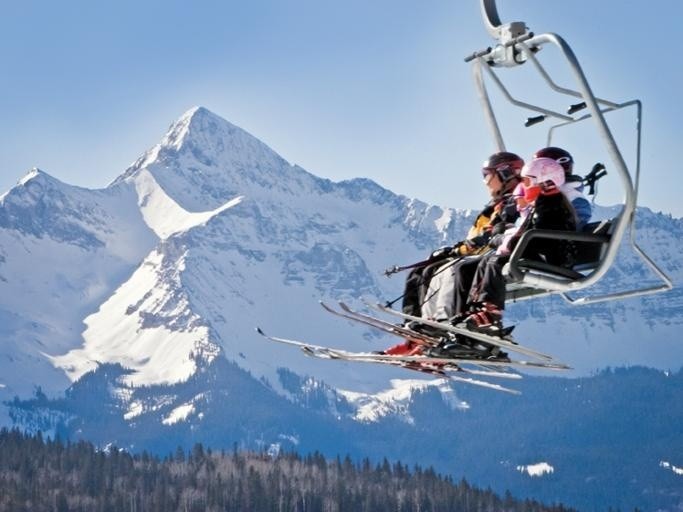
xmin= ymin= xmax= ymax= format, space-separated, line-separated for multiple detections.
xmin=517 ymin=199 xmax=529 ymax=210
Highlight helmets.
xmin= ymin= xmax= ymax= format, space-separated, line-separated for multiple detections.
xmin=482 ymin=147 xmax=573 ymax=199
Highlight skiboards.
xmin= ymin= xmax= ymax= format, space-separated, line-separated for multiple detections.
xmin=256 ymin=300 xmax=575 ymax=395
xmin=252 ymin=299 xmax=572 ymax=395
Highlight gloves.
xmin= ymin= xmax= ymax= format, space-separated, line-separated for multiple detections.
xmin=429 ymin=203 xmax=516 ymax=261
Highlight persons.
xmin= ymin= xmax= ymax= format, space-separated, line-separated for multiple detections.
xmin=380 ymin=152 xmax=521 ymax=356
xmin=464 ymin=147 xmax=598 ymax=330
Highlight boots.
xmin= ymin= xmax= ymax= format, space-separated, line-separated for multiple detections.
xmin=383 ymin=302 xmax=503 ymax=374
